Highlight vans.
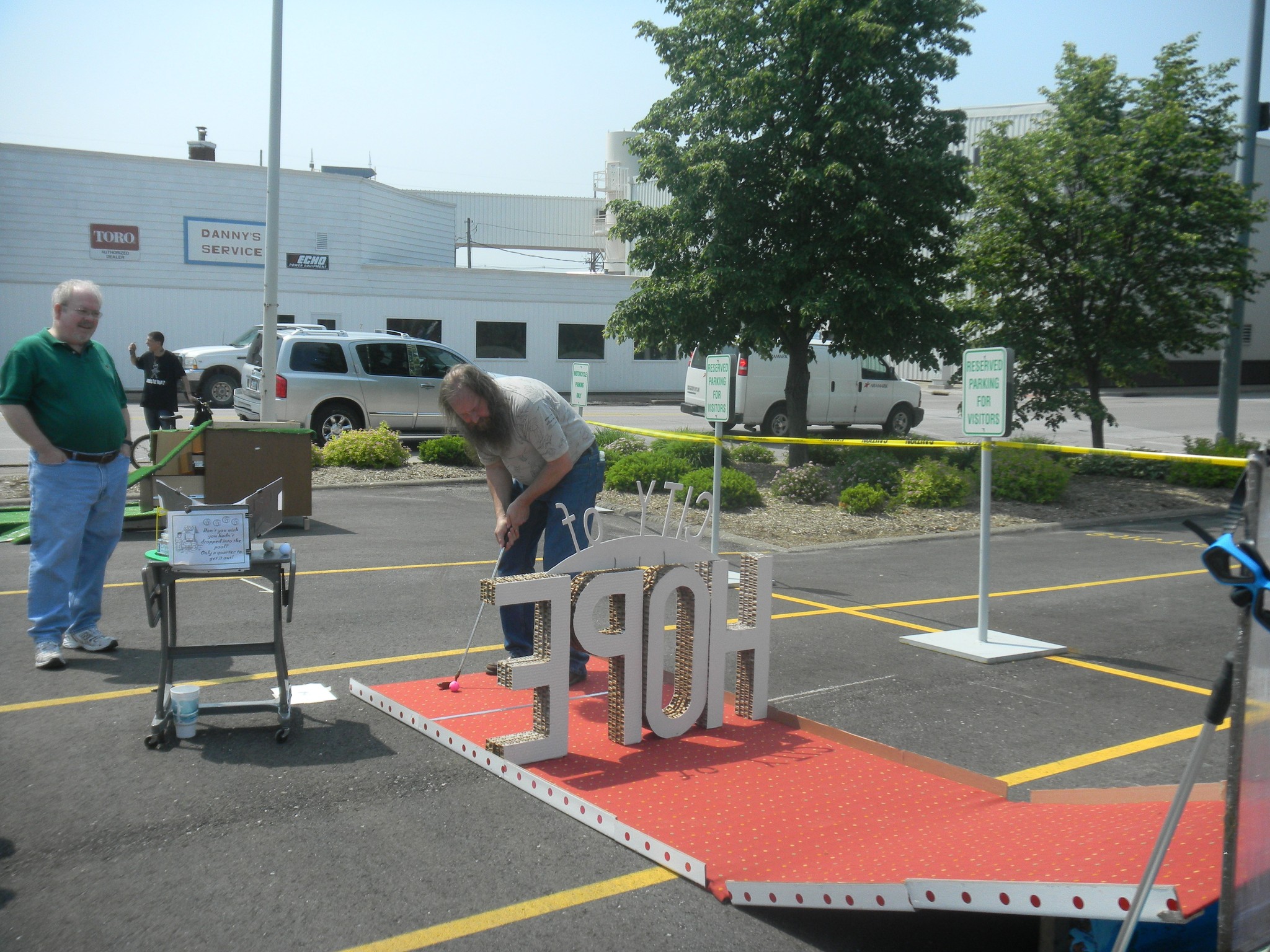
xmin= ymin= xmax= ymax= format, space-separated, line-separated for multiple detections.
xmin=680 ymin=333 xmax=925 ymax=439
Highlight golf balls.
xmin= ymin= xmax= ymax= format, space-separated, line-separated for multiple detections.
xmin=449 ymin=681 xmax=460 ymax=692
xmin=278 ymin=543 xmax=291 ymax=555
xmin=262 ymin=539 xmax=275 ymax=553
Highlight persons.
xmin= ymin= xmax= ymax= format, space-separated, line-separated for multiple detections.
xmin=127 ymin=331 xmax=197 ymax=463
xmin=0 ymin=278 xmax=131 ymax=670
xmin=437 ymin=366 xmax=605 ymax=685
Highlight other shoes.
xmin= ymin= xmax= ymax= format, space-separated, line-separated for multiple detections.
xmin=569 ymin=670 xmax=585 ymax=687
xmin=485 ymin=656 xmax=516 ymax=675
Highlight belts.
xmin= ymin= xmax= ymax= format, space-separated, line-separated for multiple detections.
xmin=61 ymin=449 xmax=122 ymax=464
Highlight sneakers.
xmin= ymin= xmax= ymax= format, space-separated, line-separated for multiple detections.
xmin=35 ymin=639 xmax=66 ymax=668
xmin=62 ymin=627 xmax=118 ymax=652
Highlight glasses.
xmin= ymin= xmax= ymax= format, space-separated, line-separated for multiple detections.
xmin=61 ymin=304 xmax=102 ymax=320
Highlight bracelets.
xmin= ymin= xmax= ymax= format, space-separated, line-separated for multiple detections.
xmin=186 ymin=392 xmax=191 ymax=395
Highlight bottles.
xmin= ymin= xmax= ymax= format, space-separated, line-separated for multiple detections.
xmin=156 ymin=528 xmax=169 ymax=556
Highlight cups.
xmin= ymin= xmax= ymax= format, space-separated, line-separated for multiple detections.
xmin=170 ymin=685 xmax=199 ymax=738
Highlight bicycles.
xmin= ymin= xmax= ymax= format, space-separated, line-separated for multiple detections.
xmin=129 ymin=395 xmax=215 ymax=472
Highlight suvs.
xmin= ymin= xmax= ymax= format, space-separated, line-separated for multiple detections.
xmin=230 ymin=327 xmax=510 ymax=447
xmin=171 ymin=323 xmax=328 ymax=410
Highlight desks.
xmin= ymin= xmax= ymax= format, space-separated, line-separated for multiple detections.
xmin=141 ymin=548 xmax=298 ymax=748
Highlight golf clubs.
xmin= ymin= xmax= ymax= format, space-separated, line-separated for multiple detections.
xmin=436 ymin=523 xmax=516 ymax=691
